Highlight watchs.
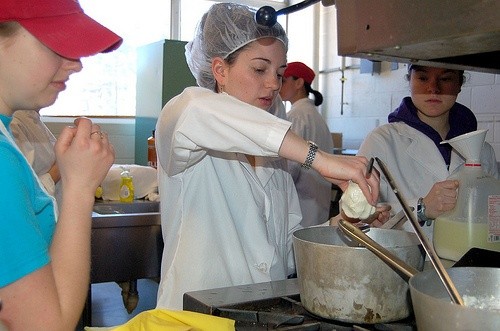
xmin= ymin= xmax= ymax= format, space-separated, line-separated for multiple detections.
xmin=417 ymin=198 xmax=432 ymax=226
xmin=301 ymin=141 xmax=319 ymax=169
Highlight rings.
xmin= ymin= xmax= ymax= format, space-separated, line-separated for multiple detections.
xmin=91 ymin=131 xmax=104 ymax=139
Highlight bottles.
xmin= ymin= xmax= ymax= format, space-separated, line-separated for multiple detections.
xmin=434 ymin=160 xmax=500 ymax=262
xmin=148 ymin=130 xmax=158 ymax=170
xmin=119 ymin=165 xmax=134 ymax=204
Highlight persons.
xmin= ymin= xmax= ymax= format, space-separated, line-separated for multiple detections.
xmin=279 ymin=62 xmax=334 ymax=227
xmin=154 ymin=2 xmax=392 ymax=311
xmin=0 ymin=0 xmax=124 ymax=330
xmin=355 ymin=61 xmax=500 ymax=260
xmin=10 ymin=108 xmax=91 ymax=330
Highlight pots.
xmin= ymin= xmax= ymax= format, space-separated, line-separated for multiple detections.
xmin=410 ymin=267 xmax=500 ymax=331
xmin=293 ymin=226 xmax=426 ymax=324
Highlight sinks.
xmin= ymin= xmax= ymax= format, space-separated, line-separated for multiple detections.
xmin=92 ymin=201 xmax=161 ymax=280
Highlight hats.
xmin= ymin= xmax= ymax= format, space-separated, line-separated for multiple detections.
xmin=284 ymin=62 xmax=316 ymax=85
xmin=0 ymin=0 xmax=123 ymax=60
